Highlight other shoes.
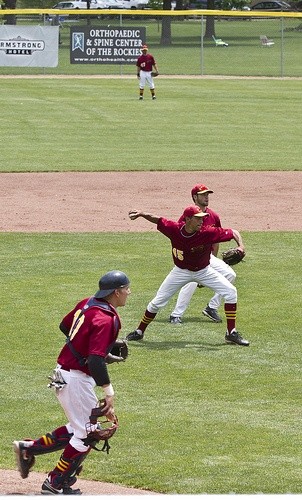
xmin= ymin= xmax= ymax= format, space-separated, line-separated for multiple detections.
xmin=139 ymin=97 xmax=142 ymax=100
xmin=152 ymin=97 xmax=156 ymax=100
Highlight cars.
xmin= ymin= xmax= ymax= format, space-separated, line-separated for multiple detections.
xmin=244 ymin=1 xmax=302 ymax=12
xmin=42 ymin=0 xmax=144 ymax=21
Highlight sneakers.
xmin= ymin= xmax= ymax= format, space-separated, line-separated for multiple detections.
xmin=14 ymin=441 xmax=34 ymax=479
xmin=202 ymin=306 xmax=222 ymax=323
xmin=41 ymin=478 xmax=81 ymax=495
xmin=170 ymin=315 xmax=182 ymax=325
xmin=225 ymin=331 xmax=249 ymax=346
xmin=124 ymin=331 xmax=143 ymax=341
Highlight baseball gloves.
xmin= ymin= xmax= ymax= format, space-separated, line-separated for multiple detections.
xmin=151 ymin=72 xmax=159 ymax=77
xmin=221 ymin=247 xmax=246 ymax=266
xmin=104 ymin=340 xmax=129 ymax=365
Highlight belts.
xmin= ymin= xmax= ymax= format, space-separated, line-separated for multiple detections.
xmin=61 ymin=366 xmax=70 ymax=371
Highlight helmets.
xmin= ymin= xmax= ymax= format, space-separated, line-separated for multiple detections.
xmin=94 ymin=270 xmax=129 ymax=298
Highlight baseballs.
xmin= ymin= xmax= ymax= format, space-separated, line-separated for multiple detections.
xmin=128 ymin=213 xmax=136 ymax=220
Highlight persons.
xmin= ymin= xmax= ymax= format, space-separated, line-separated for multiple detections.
xmin=170 ymin=184 xmax=236 ymax=324
xmin=13 ymin=270 xmax=131 ymax=495
xmin=136 ymin=45 xmax=158 ymax=100
xmin=124 ymin=205 xmax=251 ymax=347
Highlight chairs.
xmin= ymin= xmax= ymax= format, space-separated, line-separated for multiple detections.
xmin=211 ymin=34 xmax=229 ymax=48
xmin=259 ymin=34 xmax=275 ymax=47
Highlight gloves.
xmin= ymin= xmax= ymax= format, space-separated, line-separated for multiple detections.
xmin=47 ymin=369 xmax=66 ymax=391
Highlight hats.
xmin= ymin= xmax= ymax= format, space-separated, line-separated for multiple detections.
xmin=191 ymin=184 xmax=214 ymax=196
xmin=141 ymin=45 xmax=148 ymax=51
xmin=183 ymin=206 xmax=210 ymax=217
